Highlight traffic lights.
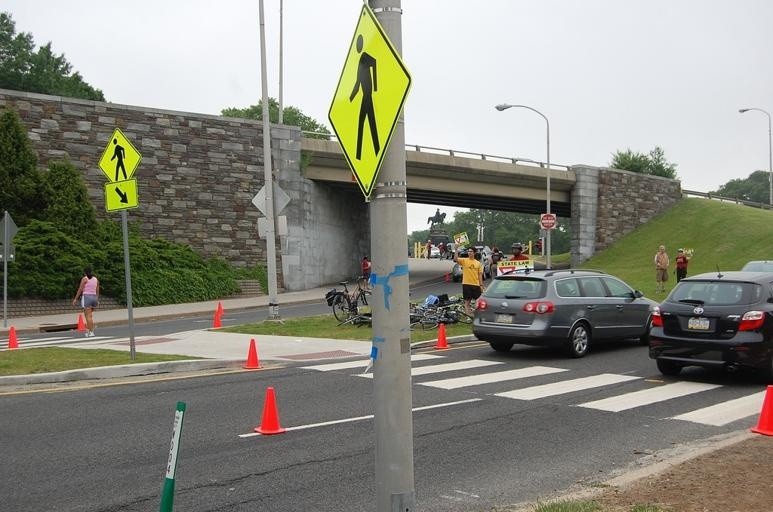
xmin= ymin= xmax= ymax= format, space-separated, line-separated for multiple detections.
xmin=534 ymin=237 xmax=544 ymax=256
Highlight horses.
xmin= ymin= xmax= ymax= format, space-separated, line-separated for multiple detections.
xmin=427 ymin=213 xmax=446 ymax=225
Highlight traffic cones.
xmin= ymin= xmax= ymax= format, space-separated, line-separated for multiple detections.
xmin=242 ymin=339 xmax=262 ymax=369
xmin=253 ymin=387 xmax=286 ymax=436
xmin=444 ymin=271 xmax=451 ymax=282
xmin=7 ymin=328 xmax=19 ymax=347
xmin=217 ymin=302 xmax=224 ymax=319
xmin=77 ymin=314 xmax=85 ymax=332
xmin=212 ymin=311 xmax=221 ymax=327
xmin=434 ymin=322 xmax=448 ymax=349
xmin=750 ymin=386 xmax=772 ymax=437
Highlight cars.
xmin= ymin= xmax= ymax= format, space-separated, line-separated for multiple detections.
xmin=427 ymin=244 xmax=502 ymax=261
xmin=740 ymin=261 xmax=773 ymax=277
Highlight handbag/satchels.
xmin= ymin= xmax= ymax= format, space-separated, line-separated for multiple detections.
xmin=326 ymin=289 xmax=341 ymax=305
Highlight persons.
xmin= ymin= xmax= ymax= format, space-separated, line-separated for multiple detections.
xmin=360 ymin=257 xmax=369 ymax=290
xmin=434 ymin=208 xmax=440 ymax=217
xmin=71 ymin=268 xmax=100 ymax=337
xmin=453 ymin=246 xmax=485 ymax=324
xmin=654 ymin=245 xmax=668 ymax=293
xmin=426 ymin=238 xmax=542 ymax=282
xmin=672 ymin=248 xmax=687 ymax=282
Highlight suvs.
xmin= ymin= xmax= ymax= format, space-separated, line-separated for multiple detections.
xmin=452 ymin=253 xmax=492 ymax=281
xmin=473 ymin=268 xmax=663 ymax=356
xmin=646 ymin=264 xmax=771 ymax=383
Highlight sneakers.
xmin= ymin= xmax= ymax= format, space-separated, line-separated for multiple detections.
xmin=85 ymin=330 xmax=95 ymax=338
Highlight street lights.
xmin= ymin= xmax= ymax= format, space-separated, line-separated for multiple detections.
xmin=739 ymin=105 xmax=772 ymax=205
xmin=475 ymin=214 xmax=480 ymax=241
xmin=480 ymin=218 xmax=485 ymax=241
xmin=495 ymin=102 xmax=552 ymax=272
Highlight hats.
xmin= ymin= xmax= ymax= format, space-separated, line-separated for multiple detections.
xmin=511 ymin=241 xmax=523 ymax=251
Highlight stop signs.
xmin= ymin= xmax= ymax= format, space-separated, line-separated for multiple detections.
xmin=540 ymin=214 xmax=556 ymax=229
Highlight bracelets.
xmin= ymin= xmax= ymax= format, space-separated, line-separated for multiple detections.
xmin=479 ymin=285 xmax=482 ymax=286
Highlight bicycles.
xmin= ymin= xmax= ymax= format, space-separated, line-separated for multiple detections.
xmin=408 ymin=291 xmax=473 ymax=331
xmin=330 ymin=277 xmax=371 ymax=329
